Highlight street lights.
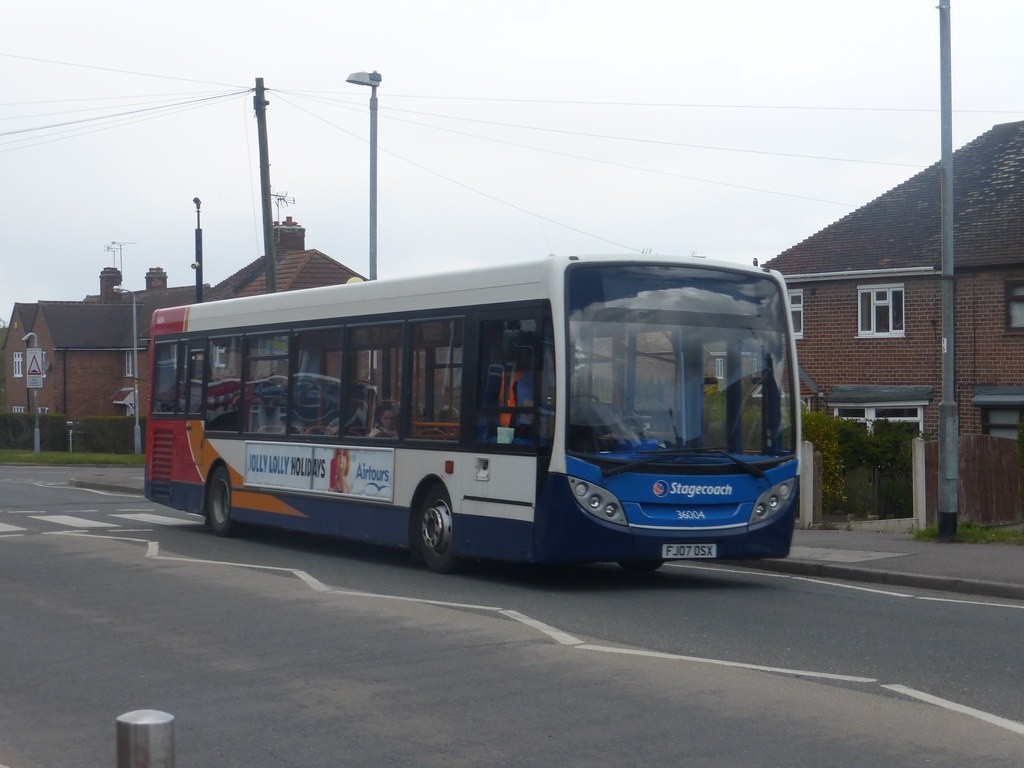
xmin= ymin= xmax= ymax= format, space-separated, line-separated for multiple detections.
xmin=346 ymin=70 xmax=382 ymax=280
xmin=113 ymin=286 xmax=141 ymax=456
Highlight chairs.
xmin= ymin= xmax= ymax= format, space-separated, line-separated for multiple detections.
xmin=257 ymin=425 xmax=447 ymax=439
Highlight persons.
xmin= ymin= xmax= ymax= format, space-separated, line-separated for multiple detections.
xmin=366 ymin=398 xmax=401 ymax=437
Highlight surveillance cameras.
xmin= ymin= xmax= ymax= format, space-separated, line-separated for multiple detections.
xmin=191 ymin=262 xmax=199 ymax=269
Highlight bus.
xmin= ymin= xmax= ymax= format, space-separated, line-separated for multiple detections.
xmin=140 ymin=252 xmax=807 ymax=578
